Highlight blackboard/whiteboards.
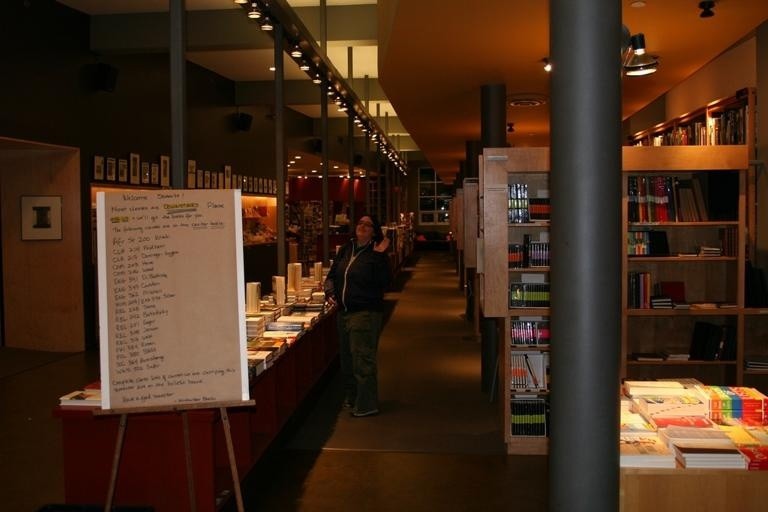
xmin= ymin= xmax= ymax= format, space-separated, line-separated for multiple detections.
xmin=92 ymin=189 xmax=256 ymax=416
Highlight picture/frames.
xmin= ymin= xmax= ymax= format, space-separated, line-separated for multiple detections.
xmin=94 ymin=152 xmax=290 ymax=196
xmin=20 ymin=194 xmax=63 ymax=242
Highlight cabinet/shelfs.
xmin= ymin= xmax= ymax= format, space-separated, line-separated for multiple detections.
xmin=449 ymin=144 xmax=768 ymax=456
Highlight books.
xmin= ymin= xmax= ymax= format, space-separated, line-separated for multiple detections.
xmin=627 ymin=271 xmax=690 ymax=309
xmin=245 ymin=265 xmax=331 ymax=379
xmin=510 ymin=350 xmax=550 ymax=393
xmin=634 ymin=348 xmax=689 ymax=362
xmin=59 ymin=390 xmax=101 ymax=406
xmin=510 ymin=281 xmax=551 ymax=308
xmin=626 ymin=224 xmax=738 ymax=258
xmin=84 ymin=381 xmax=101 ymax=390
xmin=510 ymin=183 xmax=551 ymax=224
xmin=510 ymin=320 xmax=550 ymax=344
xmin=509 ymin=234 xmax=551 ymax=269
xmin=746 ymin=358 xmax=767 ymax=369
xmin=510 ymin=395 xmax=551 ymax=438
xmin=620 ymin=377 xmax=767 ymax=471
xmin=626 ymin=176 xmax=709 ymax=223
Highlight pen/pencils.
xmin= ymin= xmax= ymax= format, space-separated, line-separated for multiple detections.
xmin=511 ymin=354 xmax=539 ymax=389
xmin=510 ymin=400 xmax=545 ymax=436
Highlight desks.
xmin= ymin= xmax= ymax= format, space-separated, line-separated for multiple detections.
xmin=51 ymin=223 xmax=415 ymax=512
xmin=619 ymin=378 xmax=768 ymax=512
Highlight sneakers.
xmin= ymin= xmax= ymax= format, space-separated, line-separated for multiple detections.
xmin=342 ymin=399 xmax=380 ymax=419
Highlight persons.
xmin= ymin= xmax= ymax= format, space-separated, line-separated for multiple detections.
xmin=322 ymin=213 xmax=405 ymax=419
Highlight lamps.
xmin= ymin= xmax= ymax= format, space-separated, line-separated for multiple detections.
xmin=542 ymin=58 xmax=552 ymax=72
xmin=621 ymin=25 xmax=661 ymax=77
